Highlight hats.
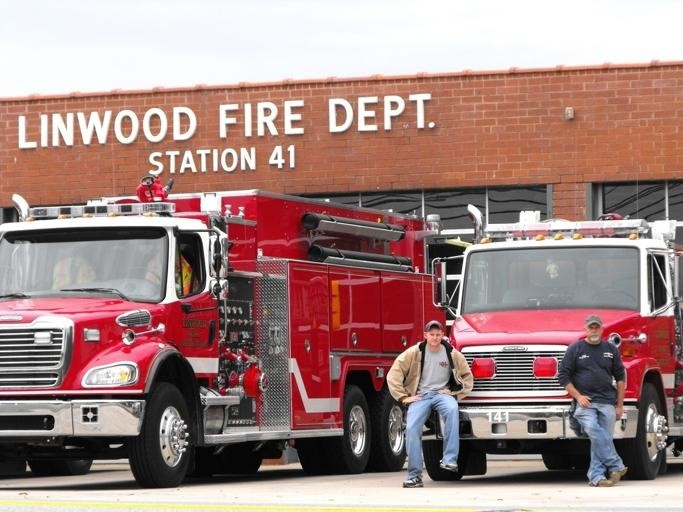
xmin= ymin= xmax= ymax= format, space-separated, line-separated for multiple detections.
xmin=425 ymin=320 xmax=442 ymax=331
xmin=585 ymin=314 xmax=602 ymax=326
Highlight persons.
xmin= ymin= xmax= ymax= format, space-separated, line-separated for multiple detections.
xmin=382 ymin=319 xmax=474 ymax=490
xmin=555 ymin=312 xmax=629 ymax=488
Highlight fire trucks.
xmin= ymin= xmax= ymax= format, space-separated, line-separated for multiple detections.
xmin=0 ymin=173 xmax=446 ymax=487
xmin=421 ymin=201 xmax=682 ymax=479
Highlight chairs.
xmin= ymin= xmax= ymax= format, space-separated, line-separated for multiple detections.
xmin=51 ymin=254 xmax=96 ymax=292
xmin=146 ymin=251 xmax=193 ymax=296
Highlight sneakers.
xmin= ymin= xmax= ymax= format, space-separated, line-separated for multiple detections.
xmin=403 ymin=477 xmax=423 ymax=488
xmin=440 ymin=461 xmax=458 ymax=471
xmin=588 ymin=467 xmax=628 ymax=486
